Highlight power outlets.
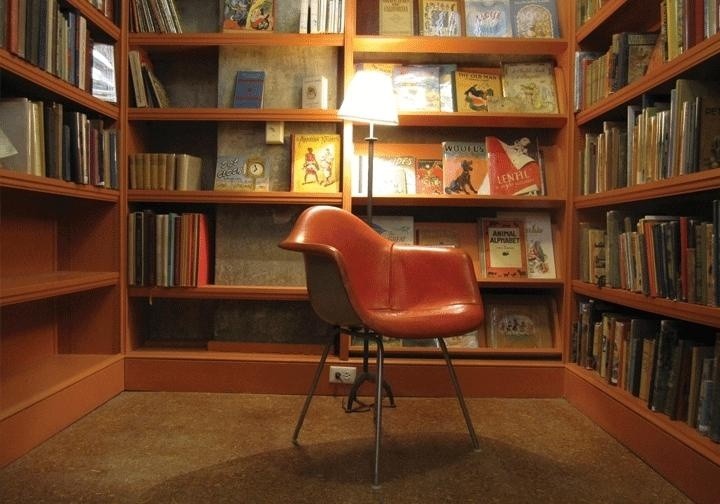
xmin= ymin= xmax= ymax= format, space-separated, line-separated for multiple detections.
xmin=328 ymin=365 xmax=359 ymax=385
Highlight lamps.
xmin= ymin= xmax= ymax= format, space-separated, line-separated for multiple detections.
xmin=334 ymin=69 xmax=403 ymax=413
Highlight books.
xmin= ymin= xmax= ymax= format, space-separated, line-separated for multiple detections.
xmin=353 ymin=56 xmax=559 ymax=350
xmin=233 ymin=70 xmax=265 ymax=110
xmin=418 ymin=0 xmax=461 ymax=36
xmin=129 ymin=49 xmax=168 ymax=109
xmin=1 ymin=1 xmax=119 ymax=190
xmin=464 ymin=0 xmax=512 ymax=37
xmin=510 ymin=0 xmax=559 ymax=39
xmin=127 ymin=210 xmax=207 ymax=287
xmin=128 ymin=121 xmax=342 ymax=195
xmin=302 ymin=76 xmax=329 ymax=109
xmin=570 ymin=1 xmax=720 ymax=448
xmin=130 ymin=1 xmax=345 ymax=33
xmin=379 ymin=0 xmax=414 ymax=36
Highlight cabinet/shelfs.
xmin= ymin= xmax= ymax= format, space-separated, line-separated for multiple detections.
xmin=121 ymin=1 xmax=568 ymax=403
xmin=564 ymin=0 xmax=720 ymax=503
xmin=0 ymin=0 xmax=123 ymax=476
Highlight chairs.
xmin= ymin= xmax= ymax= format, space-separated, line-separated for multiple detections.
xmin=274 ymin=204 xmax=487 ymax=489
